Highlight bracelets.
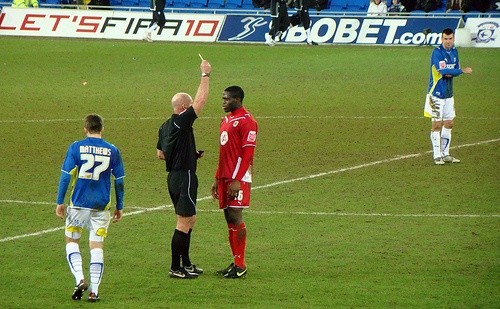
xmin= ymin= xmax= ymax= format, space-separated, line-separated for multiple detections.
xmin=202 ymin=73 xmax=210 ymax=77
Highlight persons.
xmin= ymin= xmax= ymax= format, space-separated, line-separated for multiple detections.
xmin=252 ymin=0 xmax=332 ymax=46
xmin=423 ymin=29 xmax=473 ymax=165
xmin=143 ymin=0 xmax=167 ymax=43
xmin=56 ymin=113 xmax=126 ymax=303
xmin=11 ymin=0 xmax=112 ymax=10
xmin=211 ymin=86 xmax=258 ymax=278
xmin=388 ymin=0 xmax=407 ymax=16
xmin=400 ymin=0 xmax=500 ymax=20
xmin=156 ymin=59 xmax=212 ymax=279
xmin=367 ymin=0 xmax=388 ymax=16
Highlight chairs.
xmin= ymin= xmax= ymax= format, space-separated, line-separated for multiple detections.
xmin=0 ymin=0 xmax=500 ymax=19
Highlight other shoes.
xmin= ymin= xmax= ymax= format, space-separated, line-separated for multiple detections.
xmin=307 ymin=41 xmax=318 ymax=46
xmin=434 ymin=158 xmax=445 ymax=165
xmin=265 ymin=35 xmax=276 ymax=46
xmin=142 ymin=32 xmax=153 ymax=42
xmin=443 ymin=155 xmax=460 ymax=163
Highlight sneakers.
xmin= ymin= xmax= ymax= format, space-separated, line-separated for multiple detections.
xmin=168 ymin=267 xmax=199 ymax=279
xmin=88 ymin=292 xmax=100 ymax=303
xmin=213 ymin=263 xmax=248 ymax=279
xmin=71 ymin=279 xmax=88 ymax=301
xmin=182 ymin=263 xmax=203 ymax=274
